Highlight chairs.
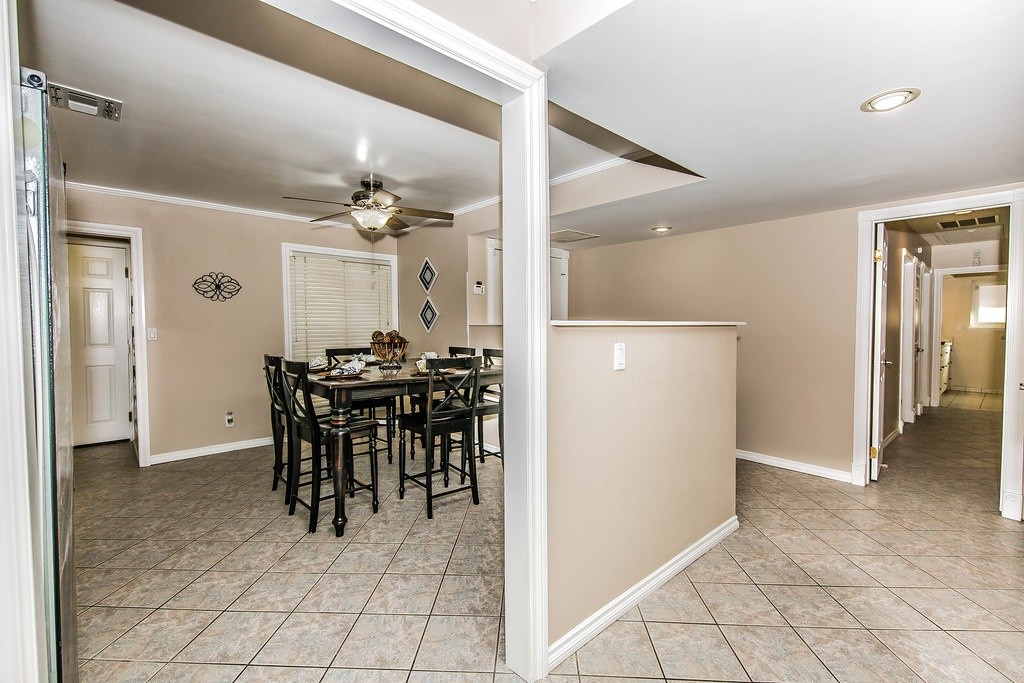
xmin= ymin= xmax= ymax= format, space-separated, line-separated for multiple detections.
xmin=263 ymin=345 xmax=503 ymax=537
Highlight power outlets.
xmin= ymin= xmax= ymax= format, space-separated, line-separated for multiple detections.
xmin=226 ymin=412 xmax=234 ymax=427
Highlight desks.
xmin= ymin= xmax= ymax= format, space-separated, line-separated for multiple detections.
xmin=287 ymin=363 xmax=503 ymax=538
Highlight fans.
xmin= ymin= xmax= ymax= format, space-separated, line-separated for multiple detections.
xmin=282 ymin=180 xmax=454 ymax=231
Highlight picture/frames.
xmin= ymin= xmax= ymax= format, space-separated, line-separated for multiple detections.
xmin=416 ymin=256 xmax=438 ymax=294
xmin=419 ymin=296 xmax=440 ymax=334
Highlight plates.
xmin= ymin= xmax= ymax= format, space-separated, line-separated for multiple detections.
xmin=317 ymin=371 xmax=364 ymax=380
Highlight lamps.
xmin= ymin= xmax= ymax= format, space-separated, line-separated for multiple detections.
xmin=350 ymin=205 xmax=398 ymax=230
